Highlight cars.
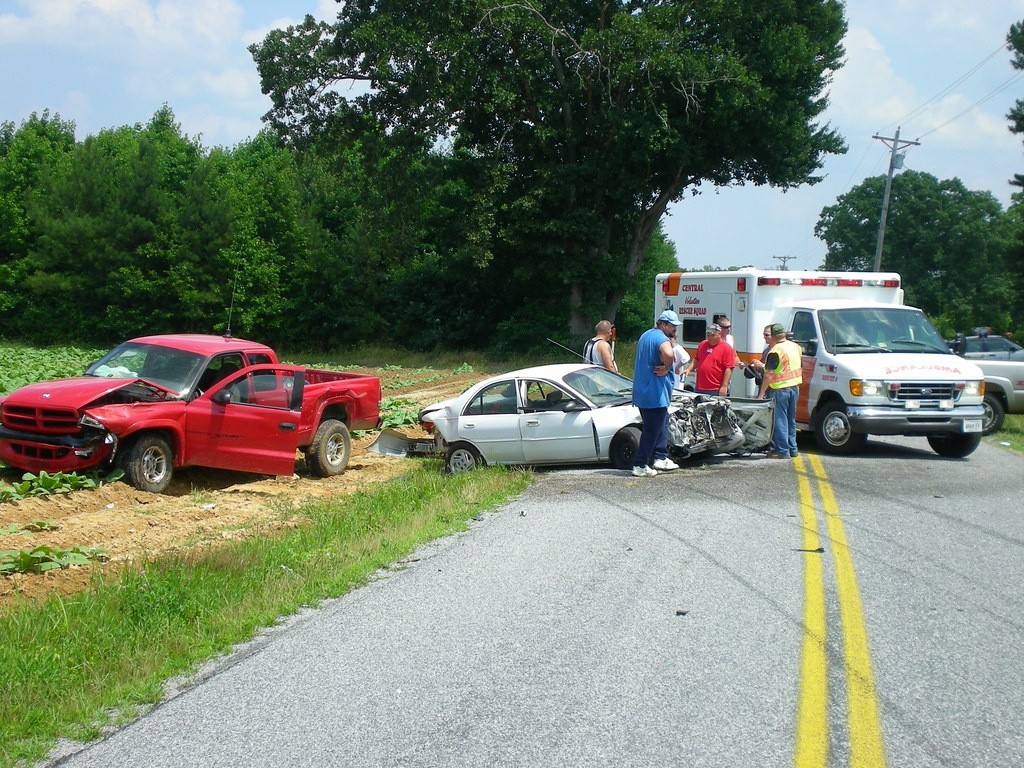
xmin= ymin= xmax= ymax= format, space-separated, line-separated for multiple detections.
xmin=948 ymin=335 xmax=1023 ymax=437
xmin=363 ymin=363 xmax=776 ymax=470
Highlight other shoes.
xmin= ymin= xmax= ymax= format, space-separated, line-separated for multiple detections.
xmin=790 ymin=452 xmax=798 ymax=457
xmin=767 ymin=451 xmax=790 ymax=459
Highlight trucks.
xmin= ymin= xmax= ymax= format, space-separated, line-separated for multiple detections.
xmin=653 ymin=266 xmax=986 ymax=460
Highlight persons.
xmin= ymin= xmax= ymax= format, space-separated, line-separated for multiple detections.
xmin=631 ymin=309 xmax=690 ymax=477
xmin=209 ymin=355 xmax=273 ymax=402
xmin=738 ymin=323 xmax=802 ymax=459
xmin=583 ymin=320 xmax=619 ymax=374
xmin=679 ymin=316 xmax=745 ymax=396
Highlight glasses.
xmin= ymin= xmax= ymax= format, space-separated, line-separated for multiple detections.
xmin=764 ymin=333 xmax=771 ymax=337
xmin=723 ymin=326 xmax=730 ymax=329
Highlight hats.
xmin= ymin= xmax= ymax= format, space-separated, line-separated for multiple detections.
xmin=659 ymin=310 xmax=683 ymax=325
xmin=595 ymin=320 xmax=614 ymax=329
xmin=771 ymin=323 xmax=784 ymax=334
xmin=706 ymin=324 xmax=722 ymax=335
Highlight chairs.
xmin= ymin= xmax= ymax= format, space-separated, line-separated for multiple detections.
xmin=546 ymin=390 xmax=567 ymax=410
xmin=210 ymin=363 xmax=242 ymax=402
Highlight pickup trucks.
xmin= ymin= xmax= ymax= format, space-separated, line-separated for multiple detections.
xmin=0 ymin=334 xmax=383 ymax=494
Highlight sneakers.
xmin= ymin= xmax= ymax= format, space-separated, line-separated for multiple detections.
xmin=632 ymin=465 xmax=657 ymax=476
xmin=653 ymin=458 xmax=679 ymax=469
